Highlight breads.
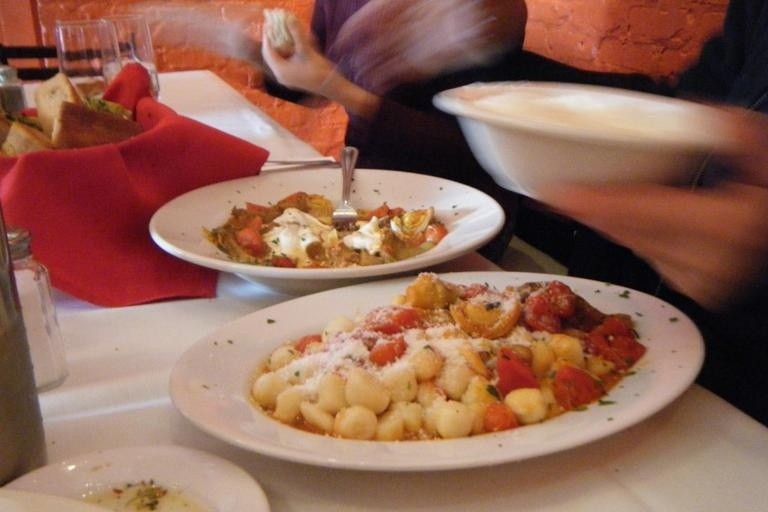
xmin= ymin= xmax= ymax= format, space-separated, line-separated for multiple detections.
xmin=262 ymin=8 xmax=296 ymax=59
xmin=0 ymin=72 xmax=141 ymax=158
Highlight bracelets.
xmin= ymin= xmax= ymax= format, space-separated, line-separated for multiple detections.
xmin=316 ymin=67 xmax=336 ymax=94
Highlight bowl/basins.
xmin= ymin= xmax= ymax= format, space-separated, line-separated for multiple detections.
xmin=431 ymin=76 xmax=756 ymax=211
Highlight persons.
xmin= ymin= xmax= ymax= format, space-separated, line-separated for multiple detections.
xmin=195 ymin=0 xmax=528 ymax=264
xmin=338 ymin=2 xmax=768 ymax=429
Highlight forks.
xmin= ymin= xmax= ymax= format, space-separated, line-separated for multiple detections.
xmin=329 ymin=143 xmax=361 ymax=232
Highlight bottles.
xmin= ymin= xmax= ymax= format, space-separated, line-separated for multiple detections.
xmin=6 ymin=227 xmax=72 ymax=395
xmin=0 ymin=64 xmax=27 ymax=115
xmin=0 ymin=200 xmax=50 ymax=486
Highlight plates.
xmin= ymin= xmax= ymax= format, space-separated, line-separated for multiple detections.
xmin=170 ymin=272 xmax=706 ymax=475
xmin=147 ymin=167 xmax=505 ymax=298
xmin=0 ymin=445 xmax=271 ymax=512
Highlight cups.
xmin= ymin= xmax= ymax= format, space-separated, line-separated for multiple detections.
xmin=55 ymin=19 xmax=128 ymax=102
xmin=95 ymin=13 xmax=160 ymax=103
